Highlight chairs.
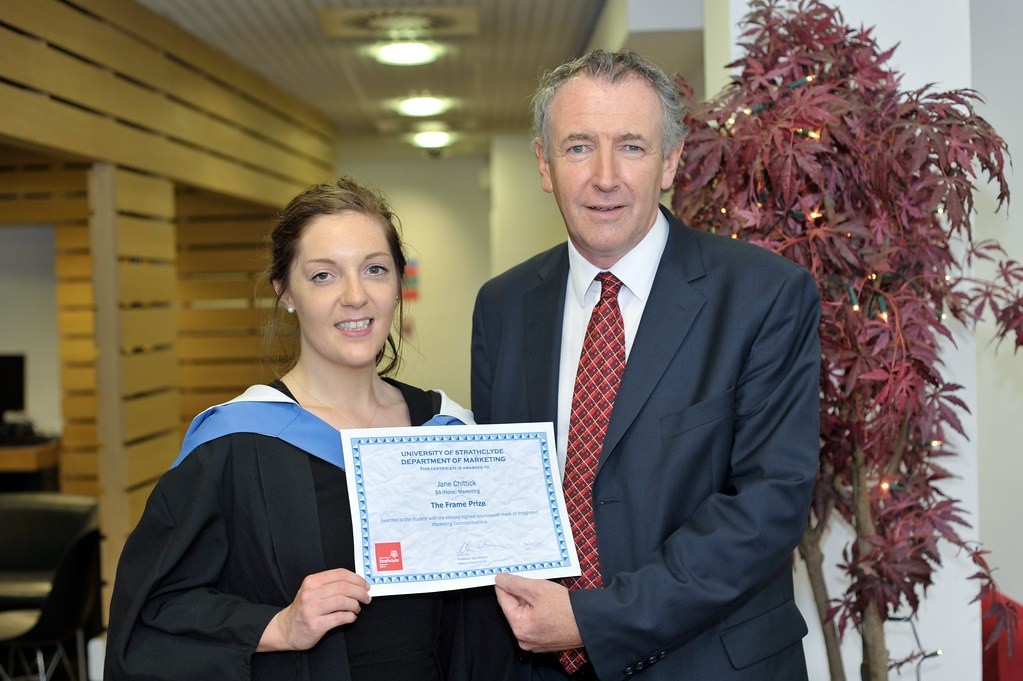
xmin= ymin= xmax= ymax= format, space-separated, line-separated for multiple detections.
xmin=0 ymin=491 xmax=97 ymax=680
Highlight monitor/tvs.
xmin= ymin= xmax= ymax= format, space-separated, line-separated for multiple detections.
xmin=0 ymin=352 xmax=35 ymax=434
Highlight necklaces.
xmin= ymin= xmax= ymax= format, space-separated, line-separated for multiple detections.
xmin=290 ymin=369 xmax=381 ymax=427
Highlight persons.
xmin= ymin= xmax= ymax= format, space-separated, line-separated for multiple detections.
xmin=103 ymin=178 xmax=520 ymax=681
xmin=469 ymin=48 xmax=821 ymax=681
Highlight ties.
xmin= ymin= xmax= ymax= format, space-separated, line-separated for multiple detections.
xmin=557 ymin=271 xmax=625 ymax=675
